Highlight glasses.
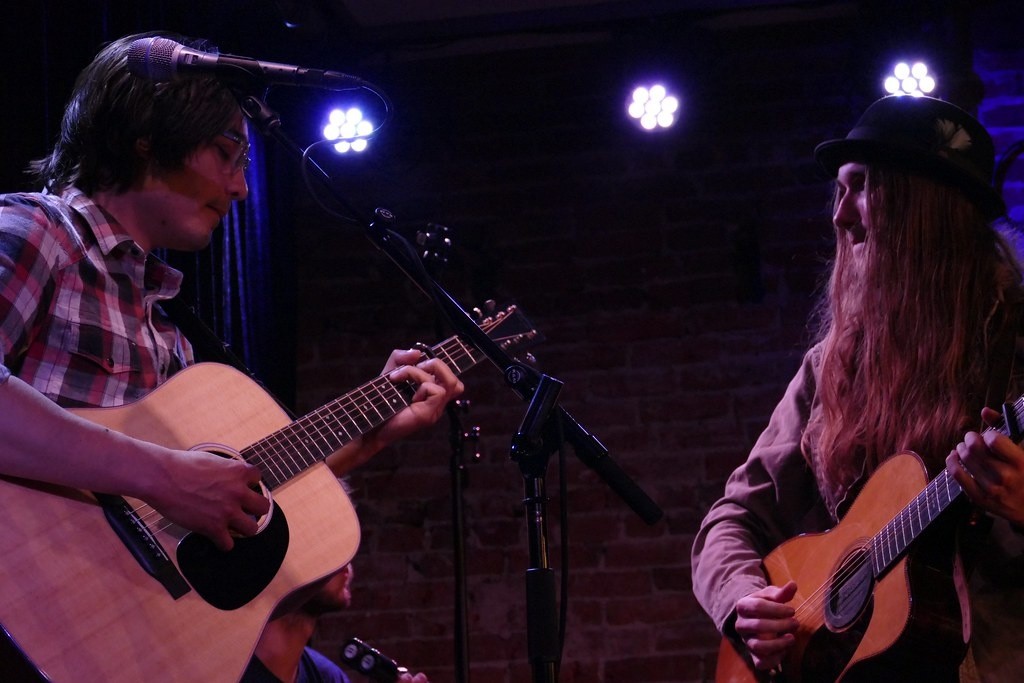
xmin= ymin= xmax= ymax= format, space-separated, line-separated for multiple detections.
xmin=219 ymin=130 xmax=252 ymax=177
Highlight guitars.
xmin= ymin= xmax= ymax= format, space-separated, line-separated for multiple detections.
xmin=713 ymin=392 xmax=1024 ymax=683
xmin=1 ymin=296 xmax=541 ymax=683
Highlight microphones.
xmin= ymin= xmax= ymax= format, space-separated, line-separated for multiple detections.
xmin=127 ymin=36 xmax=361 ymax=91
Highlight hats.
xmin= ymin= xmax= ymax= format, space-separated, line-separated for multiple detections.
xmin=814 ymin=95 xmax=1007 ymax=225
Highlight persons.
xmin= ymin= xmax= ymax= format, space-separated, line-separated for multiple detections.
xmin=230 ymin=471 xmax=432 ymax=683
xmin=687 ymin=92 xmax=1024 ymax=683
xmin=2 ymin=28 xmax=468 ymax=683
